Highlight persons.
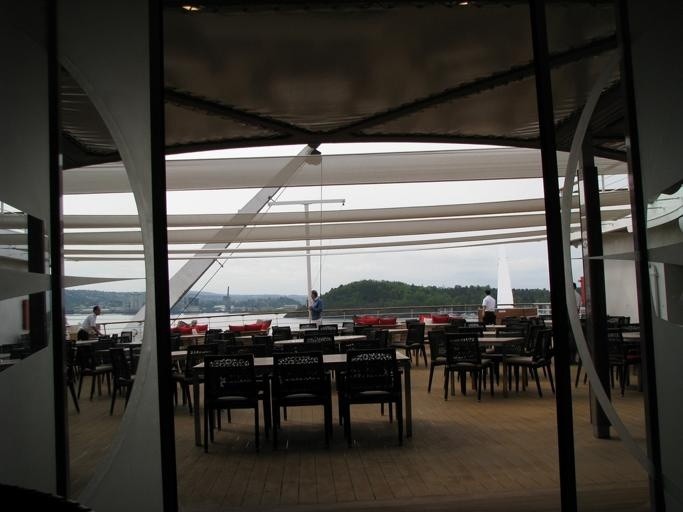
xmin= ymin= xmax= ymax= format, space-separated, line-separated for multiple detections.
xmin=571 ymin=282 xmax=582 ymax=317
xmin=481 ymin=290 xmax=496 ymax=324
xmin=190 ymin=320 xmax=200 ymax=327
xmin=76 ymin=305 xmax=104 ymax=340
xmin=176 ymin=320 xmax=188 ymax=328
xmin=306 ymin=290 xmax=324 ymax=326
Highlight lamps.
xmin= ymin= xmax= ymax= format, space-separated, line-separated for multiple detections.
xmin=305 ymin=144 xmax=322 ymax=168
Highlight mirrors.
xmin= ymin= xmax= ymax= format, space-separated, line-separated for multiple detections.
xmin=0 ymin=199 xmax=53 ymax=376
xmin=626 ymin=172 xmax=682 ymax=330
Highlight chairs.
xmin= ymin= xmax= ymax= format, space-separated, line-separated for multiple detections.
xmin=60 ymin=304 xmax=642 ymax=454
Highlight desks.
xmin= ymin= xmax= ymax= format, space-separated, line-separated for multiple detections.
xmin=194 ymin=350 xmax=415 ymax=447
xmin=179 ymin=331 xmax=206 ymax=349
xmin=452 ymin=334 xmax=525 ymax=397
xmin=228 ymin=327 xmax=268 ymax=349
xmin=375 ymin=326 xmax=409 ymax=347
xmin=69 ymin=336 xmax=113 ymax=379
xmin=273 ymin=334 xmax=367 ymax=350
xmin=602 ymin=329 xmax=641 ymax=383
xmin=289 ymin=326 xmax=349 ymax=334
